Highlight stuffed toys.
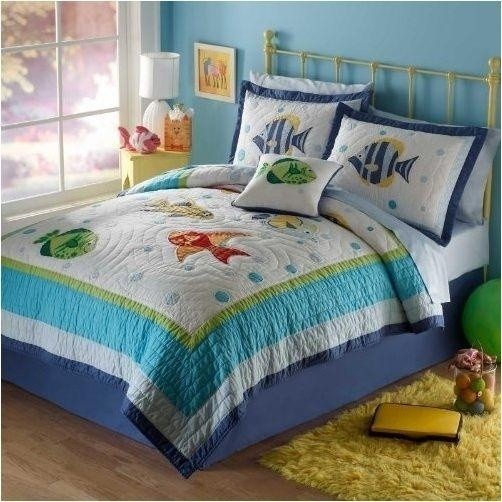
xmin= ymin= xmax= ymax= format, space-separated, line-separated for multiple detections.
xmin=117 ymin=124 xmax=162 ymax=154
xmin=452 ymin=370 xmax=495 ymax=414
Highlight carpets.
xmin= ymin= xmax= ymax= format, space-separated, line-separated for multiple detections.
xmin=255 ymin=370 xmax=501 ymax=501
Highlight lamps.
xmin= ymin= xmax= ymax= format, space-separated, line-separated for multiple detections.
xmin=137 ymin=50 xmax=181 ymax=145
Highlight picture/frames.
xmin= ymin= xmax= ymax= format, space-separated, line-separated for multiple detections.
xmin=194 ymin=41 xmax=238 ymax=105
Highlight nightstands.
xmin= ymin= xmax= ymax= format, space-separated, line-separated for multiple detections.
xmin=118 ymin=148 xmax=191 ymax=191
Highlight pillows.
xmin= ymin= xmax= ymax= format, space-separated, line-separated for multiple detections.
xmin=322 ymin=102 xmax=489 ymax=249
xmin=247 ymin=70 xmax=376 ymax=95
xmin=368 ymin=103 xmax=500 ymax=225
xmin=230 ymin=153 xmax=345 ymax=219
xmin=228 ymin=81 xmax=371 ymax=168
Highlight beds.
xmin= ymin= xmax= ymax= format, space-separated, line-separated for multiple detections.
xmin=1 ymin=29 xmax=501 ymax=473
